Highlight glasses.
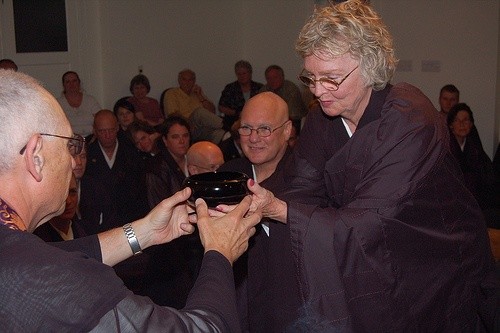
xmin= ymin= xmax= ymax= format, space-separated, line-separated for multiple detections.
xmin=236 ymin=121 xmax=287 ymax=137
xmin=20 ymin=133 xmax=86 ymax=158
xmin=454 ymin=117 xmax=472 ymax=124
xmin=296 ymin=66 xmax=358 ymax=91
xmin=193 ymin=164 xmax=219 ymax=172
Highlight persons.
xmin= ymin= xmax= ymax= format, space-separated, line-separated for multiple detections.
xmin=206 ymin=0 xmax=500 ymax=333
xmin=0 ymin=68 xmax=263 ymax=333
xmin=0 ymin=57 xmax=307 ymax=309
xmin=487 ymin=143 xmax=500 ymax=229
xmin=438 ymin=84 xmax=460 ymax=114
xmin=217 ymin=92 xmax=293 ymax=333
xmin=446 ymin=102 xmax=493 ymax=227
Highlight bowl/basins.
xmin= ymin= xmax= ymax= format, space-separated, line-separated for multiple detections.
xmin=183 ymin=170 xmax=251 ymax=207
